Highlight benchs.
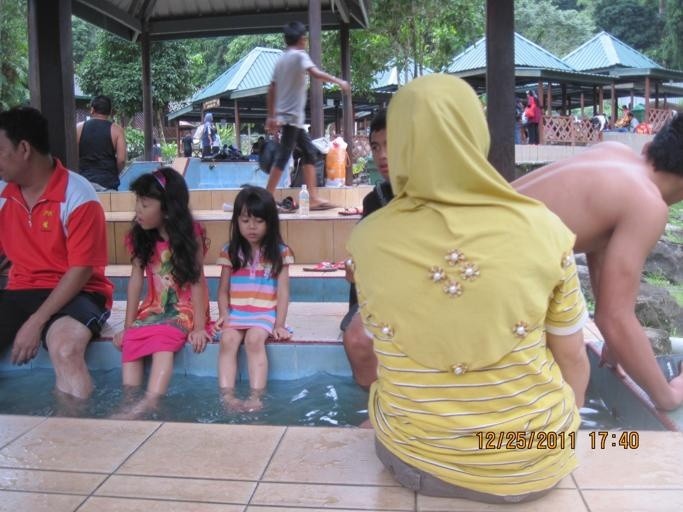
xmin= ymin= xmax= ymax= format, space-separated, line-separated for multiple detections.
xmin=543 ymin=115 xmax=602 ymax=147
xmin=648 ymin=108 xmax=673 ymax=133
xmin=329 ymin=134 xmax=372 ymax=158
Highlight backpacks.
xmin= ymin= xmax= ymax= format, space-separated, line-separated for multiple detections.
xmin=291 ymin=146 xmax=325 ymax=186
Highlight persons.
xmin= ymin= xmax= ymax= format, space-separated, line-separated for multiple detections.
xmin=208 ymin=185 xmax=296 ymax=416
xmin=179 ymin=112 xmax=264 ymax=164
xmin=513 ymin=90 xmax=523 ymax=146
xmin=337 ymin=107 xmax=395 ymax=394
xmin=151 ymin=137 xmax=161 ymax=162
xmin=523 ymin=87 xmax=541 ymax=147
xmin=517 ymin=101 xmax=530 ymax=144
xmin=75 ymin=95 xmax=127 ymax=196
xmin=542 ymin=104 xmax=653 ymax=144
xmin=343 ymin=70 xmax=591 ymax=505
xmin=0 ymin=105 xmax=115 ymax=420
xmin=507 ymin=111 xmax=683 ymax=413
xmin=106 ymin=168 xmax=214 ymax=421
xmin=263 ymin=19 xmax=351 ymax=215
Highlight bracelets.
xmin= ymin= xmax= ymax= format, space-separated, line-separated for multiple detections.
xmin=327 ymin=74 xmax=337 ymax=84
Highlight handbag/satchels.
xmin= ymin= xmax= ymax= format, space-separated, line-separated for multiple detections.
xmin=259 ymin=141 xmax=281 ymax=173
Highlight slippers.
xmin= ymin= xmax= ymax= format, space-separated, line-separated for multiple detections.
xmin=309 ymin=202 xmax=363 ymax=215
xmin=303 ymin=261 xmax=346 ymax=271
xmin=276 ymin=196 xmax=298 ymax=213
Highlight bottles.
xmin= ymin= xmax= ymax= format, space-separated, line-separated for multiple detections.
xmin=299 ymin=183 xmax=311 ymax=218
xmin=326 ymin=140 xmax=345 ymax=188
